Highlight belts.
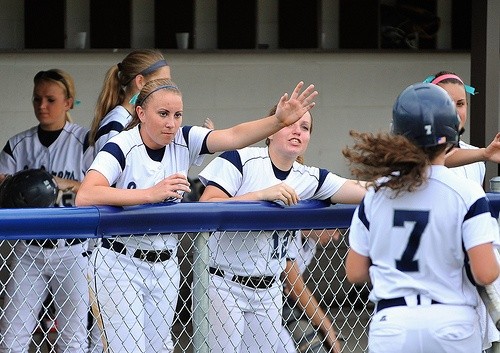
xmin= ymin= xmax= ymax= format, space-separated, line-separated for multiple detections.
xmin=101 ymin=239 xmax=172 ymax=262
xmin=210 ymin=267 xmax=276 ymax=288
xmin=26 ymin=238 xmax=87 ymax=247
xmin=377 ymin=295 xmax=439 ymax=311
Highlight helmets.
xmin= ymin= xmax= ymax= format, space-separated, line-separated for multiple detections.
xmin=0 ymin=169 xmax=57 ymax=208
xmin=393 ymin=83 xmax=460 ymax=147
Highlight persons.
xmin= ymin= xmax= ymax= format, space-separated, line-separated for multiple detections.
xmin=420 ymin=70 xmax=499 ymax=353
xmin=0 ymin=69 xmax=92 ymax=353
xmin=342 ymin=82 xmax=499 ymax=353
xmin=191 ymin=100 xmax=376 ymax=353
xmin=75 ymin=79 xmax=319 ymax=353
xmin=81 ymin=48 xmax=172 ymax=180
xmin=280 ymin=229 xmax=345 ymax=353
